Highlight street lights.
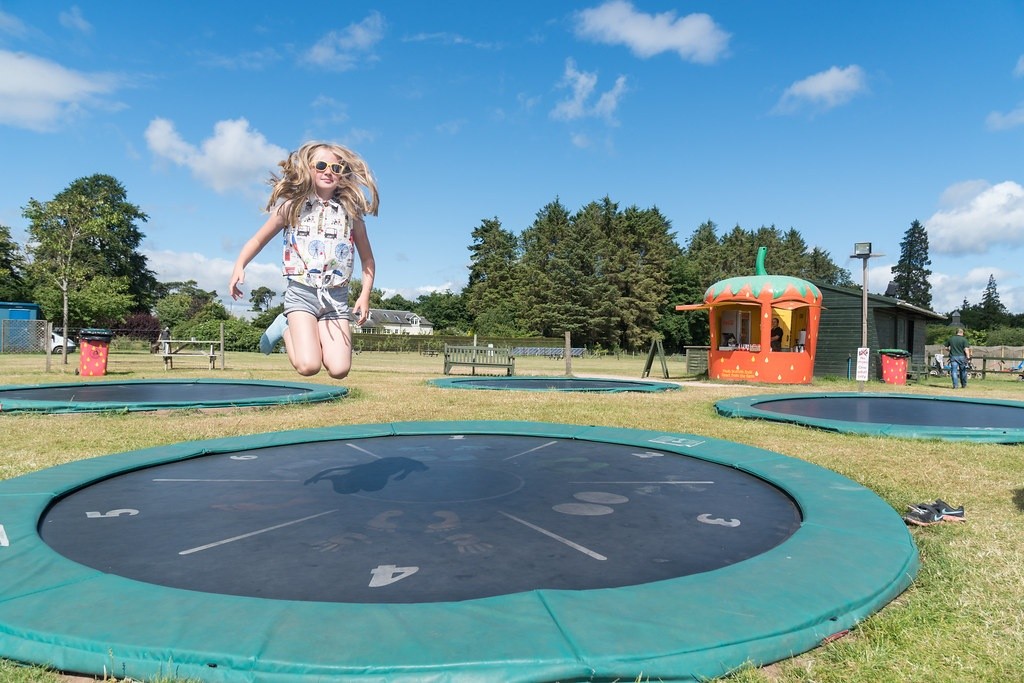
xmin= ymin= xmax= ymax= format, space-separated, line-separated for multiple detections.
xmin=853 ymin=242 xmax=873 ymax=348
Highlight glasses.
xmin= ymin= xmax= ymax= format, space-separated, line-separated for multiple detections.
xmin=309 ymin=159 xmax=346 ymax=176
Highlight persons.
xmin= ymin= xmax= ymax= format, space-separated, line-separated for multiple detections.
xmin=159 ymin=327 xmax=172 ymax=351
xmin=944 ymin=328 xmax=970 ymax=389
xmin=228 ymin=139 xmax=380 ymax=379
xmin=770 ymin=318 xmax=783 ymax=352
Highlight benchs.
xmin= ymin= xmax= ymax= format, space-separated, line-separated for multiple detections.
xmin=153 ymin=351 xmax=220 ymax=357
xmin=442 ymin=343 xmax=516 ymax=376
xmin=907 ymin=356 xmax=931 ymax=384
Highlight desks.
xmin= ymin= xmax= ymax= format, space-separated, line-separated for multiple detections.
xmin=423 ymin=349 xmax=439 ymax=357
xmin=157 ymin=339 xmax=221 ymax=371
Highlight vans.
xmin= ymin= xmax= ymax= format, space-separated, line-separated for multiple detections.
xmin=38 ymin=327 xmax=76 ymax=354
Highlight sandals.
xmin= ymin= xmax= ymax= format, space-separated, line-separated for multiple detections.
xmin=901 ymin=498 xmax=967 ymax=525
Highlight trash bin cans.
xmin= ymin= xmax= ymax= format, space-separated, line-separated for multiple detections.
xmin=79 ymin=328 xmax=113 ymax=375
xmin=878 ymin=348 xmax=912 ymax=385
